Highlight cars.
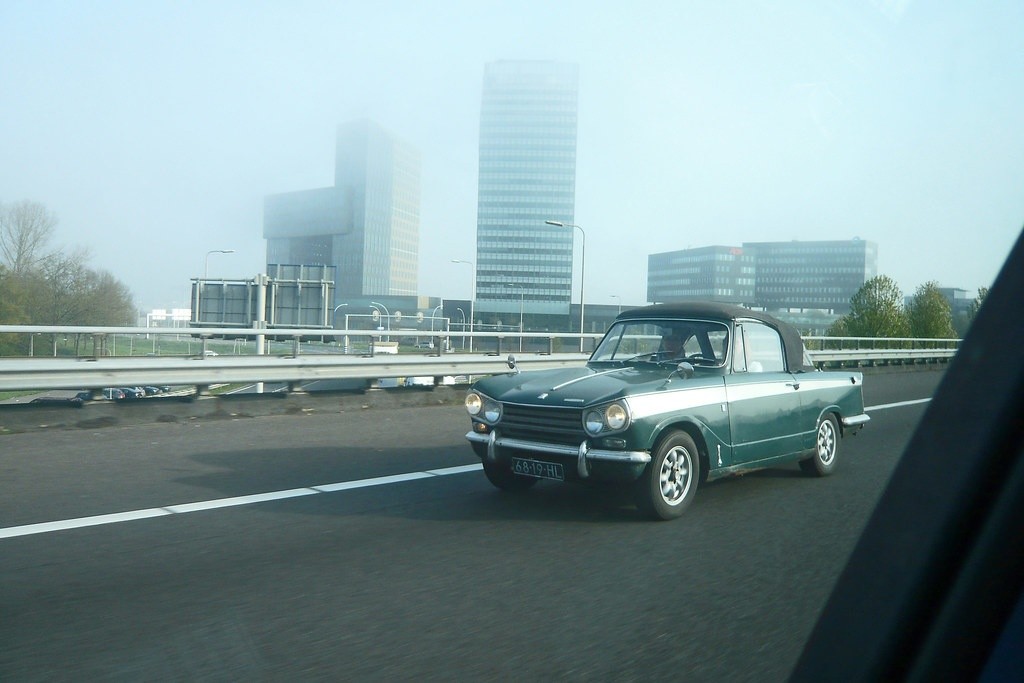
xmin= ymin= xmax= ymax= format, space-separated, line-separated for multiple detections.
xmin=28 ymin=384 xmax=171 ymax=406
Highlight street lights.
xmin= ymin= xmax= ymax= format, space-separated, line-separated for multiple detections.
xmin=429 ymin=301 xmax=466 ymax=351
xmin=334 ymin=300 xmax=390 ymax=343
xmin=448 ymin=258 xmax=478 ymax=353
xmin=544 ymin=219 xmax=585 ymax=353
xmin=509 ymin=281 xmax=525 ymax=355
xmin=205 ymin=248 xmax=235 ymax=283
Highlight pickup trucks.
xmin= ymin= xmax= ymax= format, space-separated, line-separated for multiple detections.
xmin=467 ymin=299 xmax=872 ymax=522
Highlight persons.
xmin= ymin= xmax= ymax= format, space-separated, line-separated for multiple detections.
xmin=655 ymin=336 xmax=683 ymax=360
xmin=716 ymin=334 xmax=763 ymax=373
